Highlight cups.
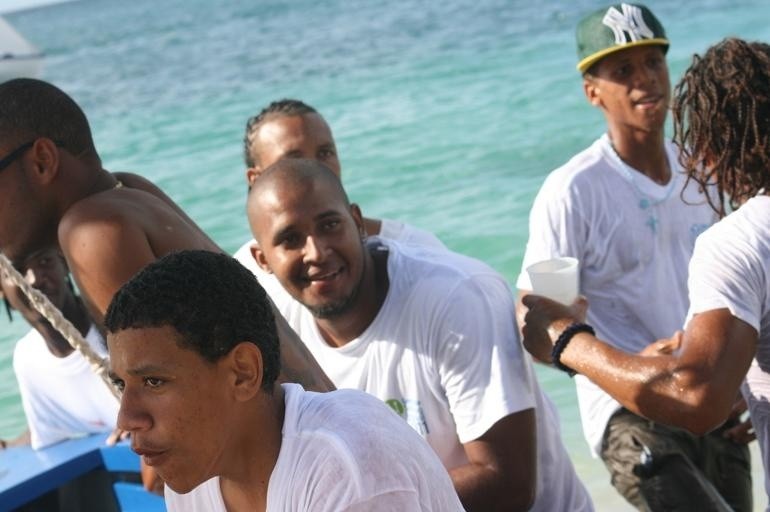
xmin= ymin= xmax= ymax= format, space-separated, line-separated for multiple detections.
xmin=526 ymin=256 xmax=581 ymax=307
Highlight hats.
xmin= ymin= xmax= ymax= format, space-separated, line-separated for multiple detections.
xmin=576 ymin=4 xmax=669 ymax=73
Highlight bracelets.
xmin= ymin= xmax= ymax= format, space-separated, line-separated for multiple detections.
xmin=550 ymin=322 xmax=596 ymax=378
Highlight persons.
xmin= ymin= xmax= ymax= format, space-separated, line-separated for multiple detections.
xmin=514 ymin=2 xmax=754 ymax=512
xmin=104 ymin=249 xmax=465 ymax=511
xmin=0 ymin=77 xmax=337 ymax=394
xmin=250 ymin=159 xmax=591 ymax=512
xmin=0 ymin=244 xmax=122 ymax=451
xmin=521 ymin=37 xmax=770 ymax=512
xmin=245 ymin=98 xmax=443 ymax=249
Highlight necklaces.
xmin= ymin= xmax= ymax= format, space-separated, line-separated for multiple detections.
xmin=114 ymin=180 xmax=123 ymax=189
